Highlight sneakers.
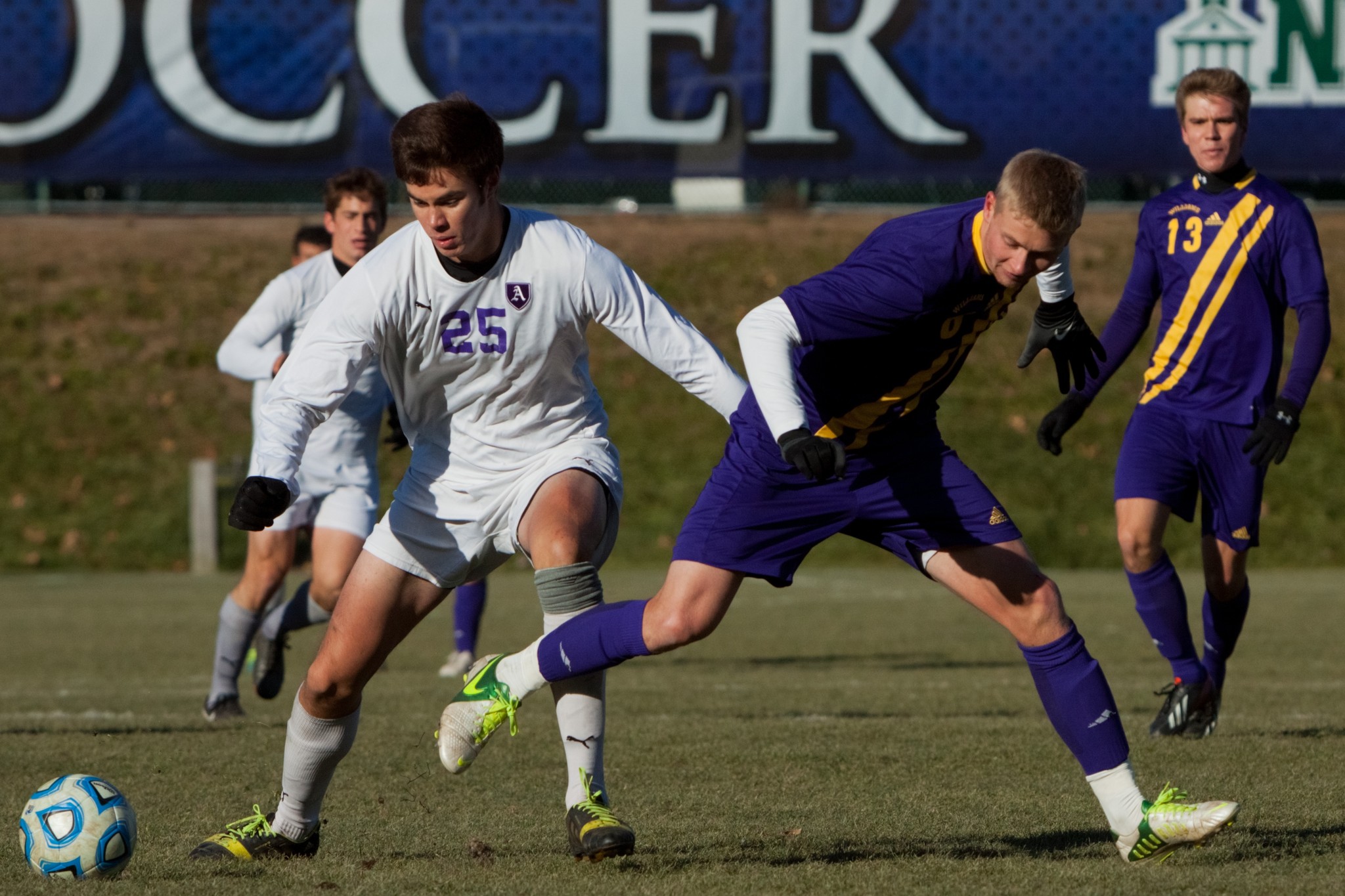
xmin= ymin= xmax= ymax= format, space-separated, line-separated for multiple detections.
xmin=187 ymin=804 xmax=321 ymax=863
xmin=565 ymin=767 xmax=635 ymax=863
xmin=1182 ymin=694 xmax=1222 ymax=740
xmin=247 ymin=621 xmax=292 ymax=700
xmin=201 ymin=693 xmax=245 ymax=722
xmin=1149 ymin=666 xmax=1219 ymax=736
xmin=434 ymin=653 xmax=522 ymax=774
xmin=1109 ymin=781 xmax=1239 ymax=865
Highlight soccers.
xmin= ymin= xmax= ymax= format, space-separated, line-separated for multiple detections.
xmin=19 ymin=772 xmax=136 ymax=879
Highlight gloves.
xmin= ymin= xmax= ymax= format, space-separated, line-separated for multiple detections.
xmin=1016 ymin=291 xmax=1107 ymax=394
xmin=1036 ymin=396 xmax=1088 ymax=456
xmin=778 ymin=427 xmax=837 ymax=479
xmin=227 ymin=477 xmax=291 ymax=532
xmin=1241 ymin=393 xmax=1302 ymax=466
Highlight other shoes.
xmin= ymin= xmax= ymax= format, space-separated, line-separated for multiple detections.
xmin=438 ymin=650 xmax=473 ymax=677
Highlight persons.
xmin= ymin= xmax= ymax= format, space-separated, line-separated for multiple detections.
xmin=437 ymin=151 xmax=1242 ymax=863
xmin=1039 ymin=67 xmax=1332 ymax=743
xmin=242 ymin=224 xmax=331 ymax=670
xmin=187 ymin=103 xmax=750 ymax=864
xmin=202 ymin=169 xmax=407 ymax=723
